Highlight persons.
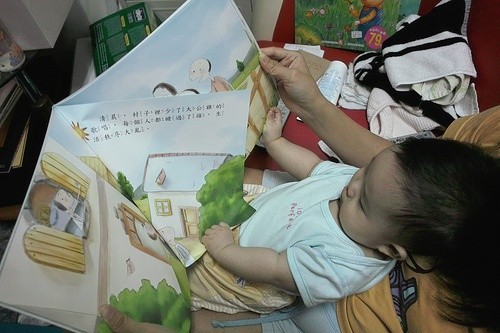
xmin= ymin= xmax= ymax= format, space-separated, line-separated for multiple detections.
xmin=98 ymin=48 xmax=500 ymax=333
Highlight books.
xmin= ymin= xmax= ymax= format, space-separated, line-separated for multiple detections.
xmin=0 ymin=0 xmax=278 ymax=333
xmin=294 ymin=0 xmax=399 ymax=53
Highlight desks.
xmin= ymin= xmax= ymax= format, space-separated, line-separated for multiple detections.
xmin=0 ymin=25 xmax=78 ymax=221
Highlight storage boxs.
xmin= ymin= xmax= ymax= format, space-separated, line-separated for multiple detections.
xmin=89 ymin=1 xmax=163 ymax=79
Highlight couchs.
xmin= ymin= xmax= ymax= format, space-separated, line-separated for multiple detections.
xmin=248 ymin=0 xmax=500 ymax=172
